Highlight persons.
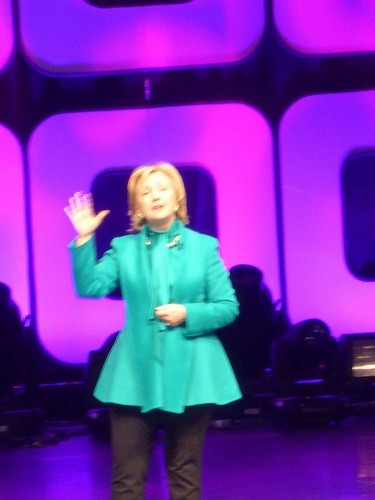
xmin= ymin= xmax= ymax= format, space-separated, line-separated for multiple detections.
xmin=64 ymin=162 xmax=243 ymax=500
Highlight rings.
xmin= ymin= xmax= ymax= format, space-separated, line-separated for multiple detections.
xmin=165 ymin=323 xmax=170 ymax=326
